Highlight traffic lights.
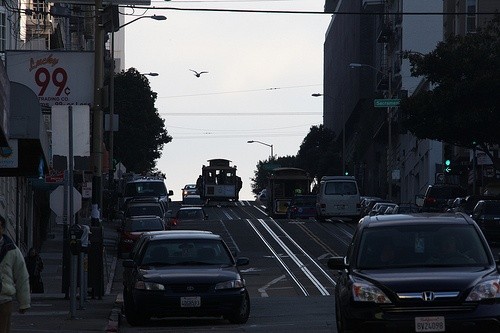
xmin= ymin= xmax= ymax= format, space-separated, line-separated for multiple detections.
xmin=445 ymin=155 xmax=452 ymax=174
xmin=344 ymin=170 xmax=349 ymax=176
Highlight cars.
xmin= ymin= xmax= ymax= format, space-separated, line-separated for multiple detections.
xmin=182 ymin=183 xmax=202 ymax=200
xmin=119 ymin=230 xmax=252 ymax=325
xmin=121 ymin=214 xmax=165 ymax=252
xmin=444 ymin=196 xmax=466 ymax=215
xmin=120 ymin=196 xmax=169 ymax=218
xmin=472 ymin=200 xmax=500 ymax=247
xmin=286 ymin=195 xmax=318 ymax=219
xmin=171 ymin=204 xmax=208 ymax=233
xmin=463 ymin=195 xmax=500 ymax=218
xmin=326 ymin=212 xmax=500 ymax=333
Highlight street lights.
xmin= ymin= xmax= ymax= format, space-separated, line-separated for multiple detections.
xmin=359 ymin=197 xmax=423 ymax=217
xmin=247 ymin=141 xmax=274 ymax=158
xmin=312 ymin=93 xmax=346 ymax=175
xmin=107 ymin=9 xmax=167 ymax=180
xmin=349 ymin=61 xmax=393 ymax=197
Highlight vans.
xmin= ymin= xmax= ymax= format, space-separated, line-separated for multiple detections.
xmin=120 ymin=178 xmax=174 ymax=210
xmin=316 ymin=176 xmax=361 ymax=223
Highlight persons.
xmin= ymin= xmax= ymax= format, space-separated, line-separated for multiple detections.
xmin=0 ymin=214 xmax=31 ymax=333
xmin=24 ymin=247 xmax=43 ymax=294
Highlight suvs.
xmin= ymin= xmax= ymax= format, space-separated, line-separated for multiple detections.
xmin=416 ymin=184 xmax=466 ymax=215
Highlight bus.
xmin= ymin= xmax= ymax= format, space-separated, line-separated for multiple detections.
xmin=266 ymin=167 xmax=311 ymax=219
xmin=201 ymin=158 xmax=241 ymax=202
